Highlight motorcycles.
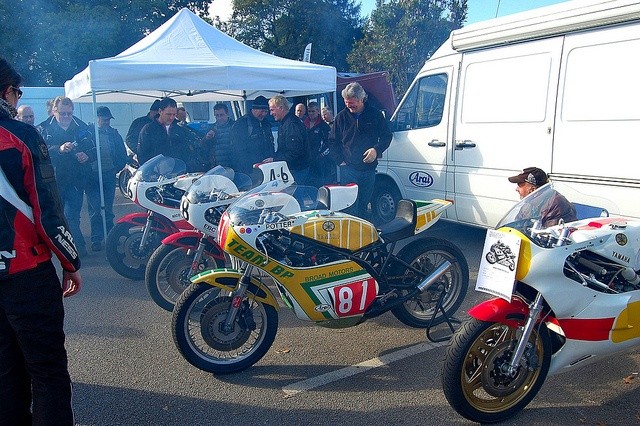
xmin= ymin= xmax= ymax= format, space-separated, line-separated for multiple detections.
xmin=144 ymin=164 xmax=359 ymax=312
xmin=105 ymin=153 xmax=295 ymax=280
xmin=441 ymin=179 xmax=640 ymax=423
xmin=170 ymin=178 xmax=470 ymax=374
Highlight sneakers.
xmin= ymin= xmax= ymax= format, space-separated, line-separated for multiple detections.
xmin=78 ymin=245 xmax=88 ymax=256
xmin=91 ymin=240 xmax=102 ymax=251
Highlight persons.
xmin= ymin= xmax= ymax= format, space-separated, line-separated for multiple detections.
xmin=168 ymin=107 xmax=199 ymax=134
xmin=261 ymin=95 xmax=312 ymax=185
xmin=46 ymin=99 xmax=54 ymax=117
xmin=84 ymin=105 xmax=128 ymax=252
xmin=199 ymin=103 xmax=236 ymax=169
xmin=0 ymin=56 xmax=83 ymax=425
xmin=321 ymin=105 xmax=336 ymax=129
xmin=41 ymin=96 xmax=96 ymax=258
xmin=228 ymin=95 xmax=275 ymax=170
xmin=137 ymin=98 xmax=184 ymax=166
xmin=17 ymin=105 xmax=35 ymax=126
xmin=294 ymin=103 xmax=307 ymax=123
xmin=125 ymin=99 xmax=161 ymax=154
xmin=507 ymin=166 xmax=578 ymax=228
xmin=304 ymin=102 xmax=331 ymax=186
xmin=327 ymin=80 xmax=393 ymax=219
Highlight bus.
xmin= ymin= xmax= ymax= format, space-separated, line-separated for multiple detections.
xmin=13 ymin=87 xmax=289 ymax=193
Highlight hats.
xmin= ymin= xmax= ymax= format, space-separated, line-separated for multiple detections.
xmin=97 ymin=105 xmax=115 ymax=119
xmin=150 ymin=99 xmax=160 ymax=110
xmin=252 ymin=95 xmax=269 ymax=109
xmin=508 ymin=166 xmax=549 ymax=185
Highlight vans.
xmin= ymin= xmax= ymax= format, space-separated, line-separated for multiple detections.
xmin=371 ymin=1 xmax=640 ymax=228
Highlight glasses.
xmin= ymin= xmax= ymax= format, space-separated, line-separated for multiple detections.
xmin=7 ymin=85 xmax=23 ymax=98
xmin=55 ymin=109 xmax=74 ymax=116
